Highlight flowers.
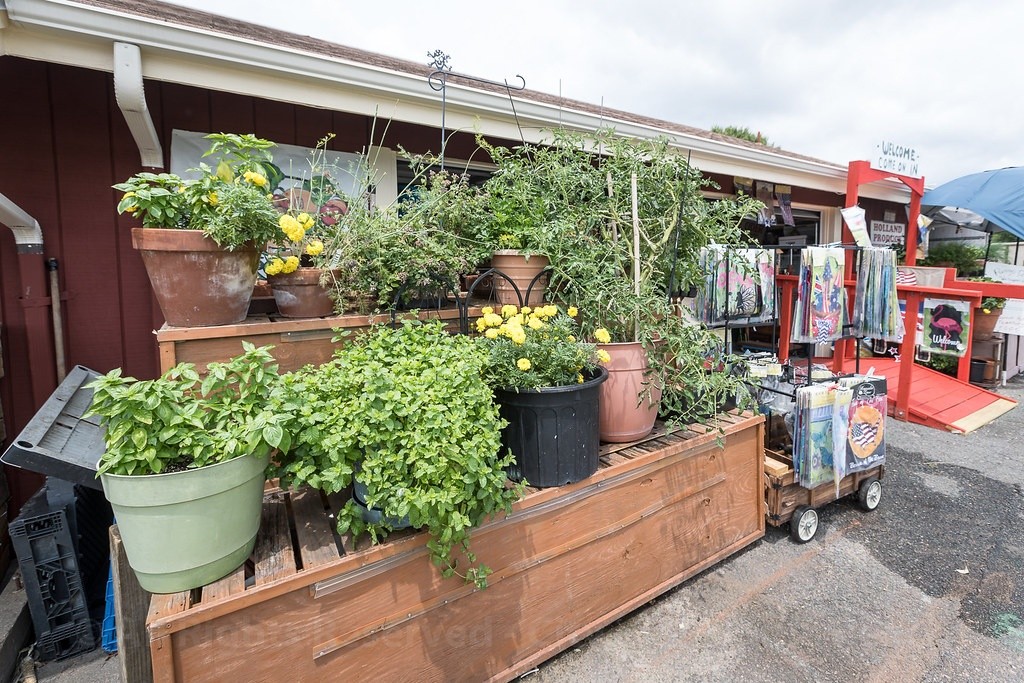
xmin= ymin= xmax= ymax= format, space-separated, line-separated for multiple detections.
xmin=109 ymin=130 xmax=324 ymax=274
xmin=474 ymin=300 xmax=612 ymax=384
xmin=965 ymin=276 xmax=1006 ymax=314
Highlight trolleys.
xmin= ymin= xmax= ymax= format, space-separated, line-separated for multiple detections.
xmin=765 ymin=421 xmax=885 ymax=544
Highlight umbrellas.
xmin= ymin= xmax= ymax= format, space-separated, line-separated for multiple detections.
xmin=908 ymin=166 xmax=1024 ymax=243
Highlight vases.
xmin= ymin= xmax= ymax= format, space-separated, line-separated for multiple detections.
xmin=484 ymin=362 xmax=613 ymax=488
xmin=972 ymin=309 xmax=1003 ymax=341
xmin=129 ymin=226 xmax=266 ymax=331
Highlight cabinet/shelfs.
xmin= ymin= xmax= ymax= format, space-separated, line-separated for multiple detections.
xmin=146 ymin=296 xmax=768 ymax=683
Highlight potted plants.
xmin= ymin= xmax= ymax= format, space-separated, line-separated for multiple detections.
xmin=520 ymin=125 xmax=766 ymax=444
xmin=474 ymin=133 xmax=555 ymax=307
xmin=269 ymin=134 xmax=380 ymax=318
xmin=275 ymin=318 xmax=522 ymax=591
xmin=80 ymin=339 xmax=297 ymax=596
